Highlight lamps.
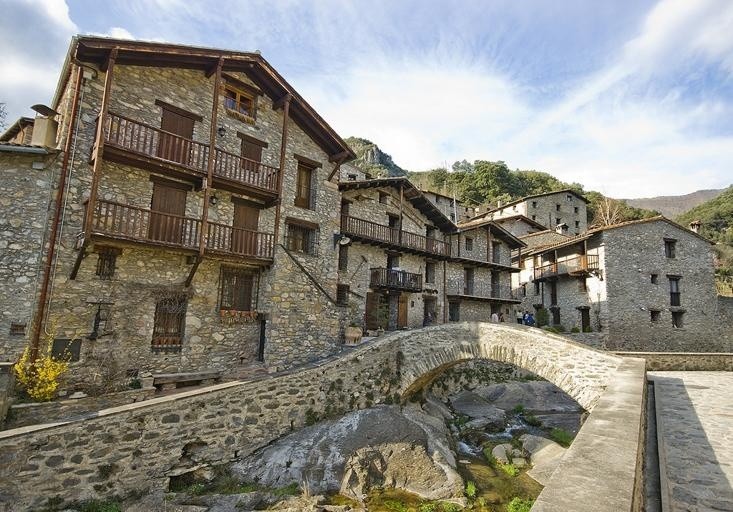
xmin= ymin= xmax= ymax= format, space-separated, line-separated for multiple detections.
xmin=218 ymin=125 xmax=226 ymax=137
xmin=333 ymin=233 xmax=351 ymax=251
xmin=210 ymin=193 xmax=218 ymax=205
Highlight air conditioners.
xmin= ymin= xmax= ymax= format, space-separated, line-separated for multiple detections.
xmin=48 ymin=337 xmax=88 ymax=367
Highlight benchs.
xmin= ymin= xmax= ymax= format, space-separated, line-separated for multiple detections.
xmin=151 ymin=370 xmax=221 ymax=391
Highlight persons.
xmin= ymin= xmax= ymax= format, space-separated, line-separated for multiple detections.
xmin=490 ymin=311 xmax=505 ymax=322
xmin=516 ymin=309 xmax=536 ymax=327
